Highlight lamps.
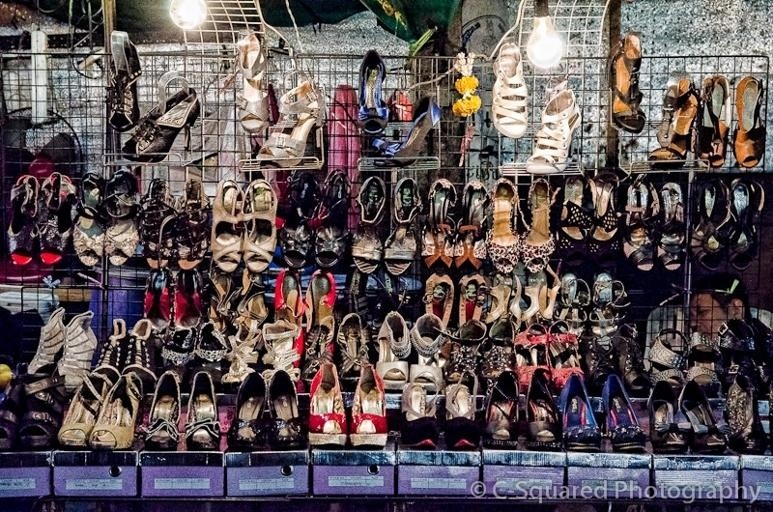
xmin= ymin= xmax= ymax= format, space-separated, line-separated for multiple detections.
xmin=526 ymin=2 xmax=564 ymax=70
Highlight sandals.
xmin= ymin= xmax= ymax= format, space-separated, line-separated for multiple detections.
xmin=209 ymin=272 xmax=233 ymax=329
xmin=516 ymin=322 xmax=555 ymax=384
xmin=550 ymin=328 xmax=585 ymax=386
xmin=195 ymin=318 xmax=225 ymax=381
xmin=241 ymin=180 xmax=279 ymax=271
xmin=585 ymin=333 xmax=617 ymax=395
xmin=382 ymin=178 xmax=421 ymax=276
xmin=688 ymin=178 xmax=729 ymax=270
xmin=652 ymin=327 xmax=686 ymax=394
xmin=590 ymin=172 xmax=623 ymax=263
xmin=173 ymin=270 xmax=206 ymax=323
xmin=222 ymin=318 xmax=263 ymax=383
xmin=488 ymin=178 xmax=523 ymax=274
xmin=339 ymin=313 xmax=371 ymax=381
xmin=459 ymin=272 xmax=487 ymax=327
xmin=312 ymin=172 xmax=349 ymax=267
xmin=236 ymin=270 xmax=268 ymax=328
xmin=491 ymin=45 xmax=527 ymax=133
xmin=210 ymin=178 xmax=244 ymax=270
xmin=487 ymin=274 xmax=512 ymax=325
xmin=455 ymin=179 xmax=487 ymax=270
xmin=262 ymin=321 xmax=301 ymax=385
xmin=72 ymin=173 xmax=103 ymax=268
xmin=721 ymin=318 xmax=763 ymax=388
xmin=39 ymin=172 xmax=74 ymax=266
xmin=689 ymin=330 xmax=722 ymax=397
xmin=525 ymin=274 xmax=559 ymax=334
xmin=655 ymin=178 xmax=687 ymax=270
xmin=624 ymin=176 xmax=655 ymax=272
xmin=732 ymin=75 xmax=768 ymax=167
xmin=525 ymin=89 xmax=582 ymax=174
xmin=589 ymin=273 xmax=628 ymax=335
xmin=8 ymin=174 xmax=38 ymax=268
xmin=445 ymin=322 xmax=483 ymax=386
xmin=141 ymin=269 xmax=171 ymax=320
xmin=175 ymin=178 xmax=207 ymax=271
xmin=729 ymin=179 xmax=765 ymax=270
xmin=423 ymin=180 xmax=458 ymax=273
xmin=141 ymin=179 xmax=173 ymax=267
xmin=554 ymin=274 xmax=589 ymax=334
xmin=305 ymin=316 xmax=337 ymax=386
xmin=556 ymin=179 xmax=589 ymax=265
xmin=421 ymin=274 xmax=454 ymax=329
xmin=509 ymin=273 xmax=522 ymax=325
xmin=271 ymin=267 xmax=305 ymax=367
xmin=371 ymin=309 xmax=412 ymax=390
xmin=617 ymin=333 xmax=649 ymax=400
xmin=162 ymin=320 xmax=195 ymax=369
xmin=483 ymin=322 xmax=520 ymax=380
xmin=408 ymin=315 xmax=445 ymax=392
xmin=524 ymin=181 xmax=556 ymax=272
xmin=700 ymin=75 xmax=730 ymax=167
xmin=607 ymin=35 xmax=646 ymax=133
xmin=648 ymin=78 xmax=700 ymax=170
xmin=350 ymin=177 xmax=387 ymax=274
xmin=307 ymin=270 xmax=336 ymax=363
xmin=104 ymin=168 xmax=139 ymax=268
xmin=280 ymin=178 xmax=319 ymax=266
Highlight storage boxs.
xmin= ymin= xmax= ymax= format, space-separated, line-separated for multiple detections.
xmin=395 ymin=447 xmax=483 ymax=495
xmin=653 ymin=451 xmax=739 ymax=502
xmin=569 ymin=451 xmax=654 ymax=499
xmin=222 ymin=448 xmax=314 ymax=497
xmin=49 ymin=449 xmax=140 ymax=497
xmin=741 ymin=453 xmax=773 ymax=506
xmin=0 ymin=449 xmax=52 ymax=502
xmin=139 ymin=449 xmax=228 ymax=495
xmin=481 ymin=446 xmax=568 ymax=499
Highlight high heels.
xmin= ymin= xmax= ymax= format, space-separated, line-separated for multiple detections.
xmin=143 ymin=371 xmax=180 ymax=451
xmin=0 ymin=375 xmax=15 ymax=450
xmin=25 ymin=307 xmax=65 ymax=375
xmin=90 ymin=319 xmax=130 ymax=379
xmin=266 ymin=370 xmax=303 ymax=449
xmin=122 ymin=86 xmax=202 ymax=162
xmin=373 ymin=97 xmax=442 ymax=167
xmin=16 ymin=373 xmax=60 ymax=449
xmin=106 ymin=40 xmax=142 ymax=131
xmin=358 ymin=51 xmax=388 ymax=137
xmin=480 ymin=371 xmax=519 ymax=447
xmin=680 ymin=380 xmax=726 ymax=456
xmin=562 ymin=373 xmax=603 ymax=450
xmin=183 ymin=371 xmax=219 ymax=450
xmin=14 ymin=309 xmax=43 ymax=373
xmin=89 ymin=372 xmax=142 ymax=449
xmin=307 ymin=362 xmax=345 ymax=448
xmin=119 ymin=319 xmax=155 ymax=383
xmin=400 ymin=375 xmax=440 ymax=450
xmin=227 ymin=371 xmax=266 ymax=451
xmin=524 ymin=368 xmax=561 ymax=449
xmin=56 ymin=310 xmax=99 ymax=393
xmin=648 ymin=382 xmax=687 ymax=455
xmin=442 ymin=372 xmax=478 ymax=449
xmin=601 ymin=374 xmax=646 ymax=452
xmin=727 ymin=373 xmax=766 ymax=455
xmin=56 ymin=372 xmax=114 ymax=449
xmin=234 ymin=33 xmax=267 ymax=130
xmin=349 ymin=364 xmax=388 ymax=446
xmin=248 ymin=81 xmax=325 ymax=165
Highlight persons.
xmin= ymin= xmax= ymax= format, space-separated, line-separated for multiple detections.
xmin=690 ymin=274 xmax=772 ymax=338
